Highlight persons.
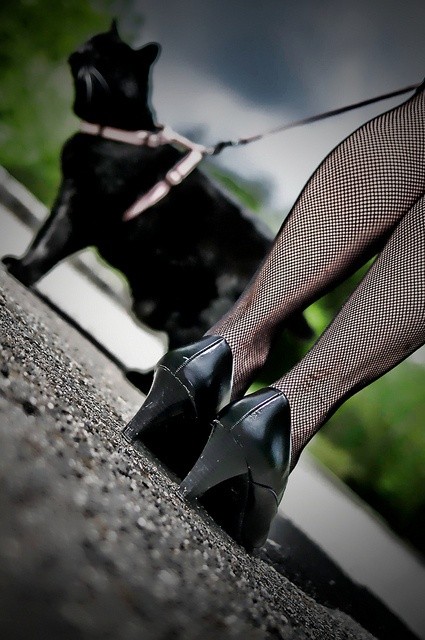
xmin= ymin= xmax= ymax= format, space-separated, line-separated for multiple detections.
xmin=115 ymin=84 xmax=425 ymax=555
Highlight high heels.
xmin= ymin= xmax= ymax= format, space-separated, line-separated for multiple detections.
xmin=121 ymin=335 xmax=234 ymax=484
xmin=179 ymin=387 xmax=291 ymax=555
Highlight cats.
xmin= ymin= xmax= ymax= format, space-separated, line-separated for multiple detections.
xmin=1 ymin=15 xmax=317 ymax=398
